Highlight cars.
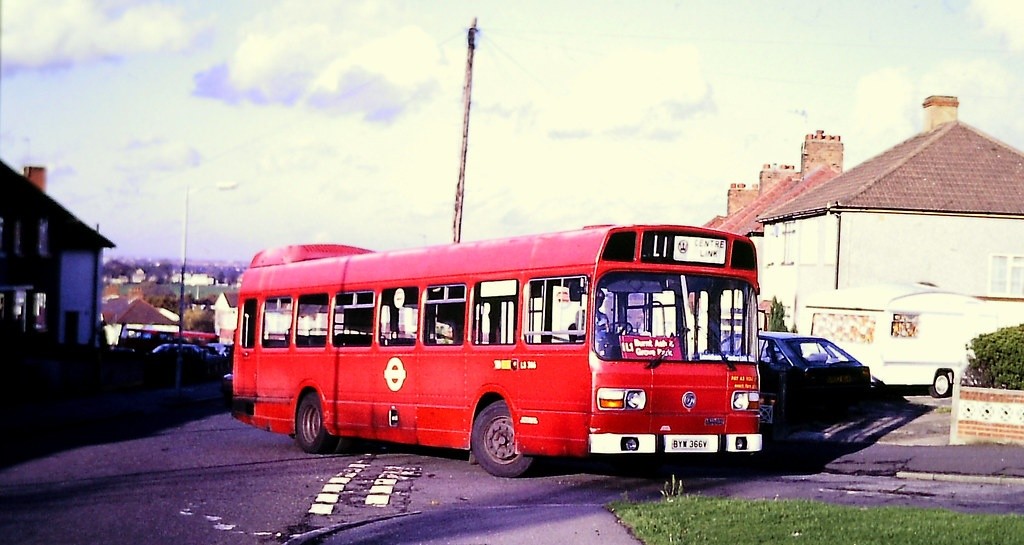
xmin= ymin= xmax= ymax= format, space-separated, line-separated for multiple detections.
xmin=720 ymin=332 xmax=870 ymax=421
xmin=115 ymin=342 xmax=234 ymax=377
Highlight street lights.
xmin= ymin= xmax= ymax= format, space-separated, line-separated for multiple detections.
xmin=178 ymin=181 xmax=237 ymax=338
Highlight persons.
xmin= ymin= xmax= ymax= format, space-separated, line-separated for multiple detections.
xmin=574 ymin=289 xmax=610 ymax=343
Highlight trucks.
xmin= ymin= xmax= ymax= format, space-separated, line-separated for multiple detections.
xmin=804 ymin=286 xmax=996 ymax=399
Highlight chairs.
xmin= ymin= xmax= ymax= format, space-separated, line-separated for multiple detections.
xmin=568 ymin=324 xmax=587 ymax=345
xmin=282 ymin=324 xmax=438 ymax=348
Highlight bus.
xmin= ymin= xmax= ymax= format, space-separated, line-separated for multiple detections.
xmin=230 ymin=223 xmax=762 ymax=478
xmin=119 ymin=327 xmax=220 ymax=348
